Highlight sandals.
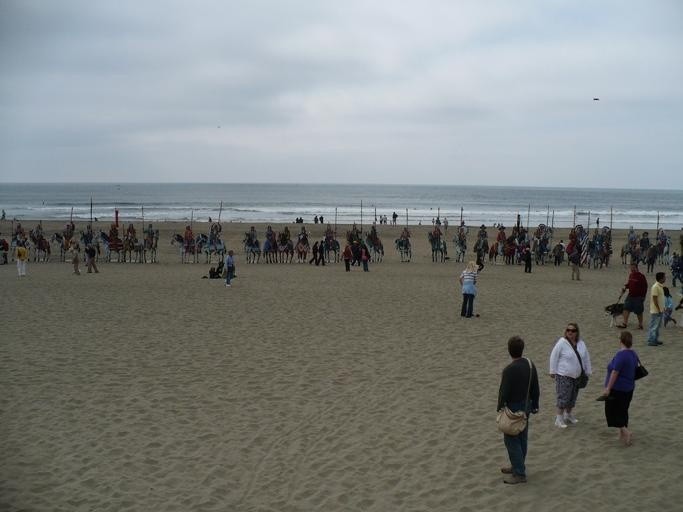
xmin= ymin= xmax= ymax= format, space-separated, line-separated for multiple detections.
xmin=636 ymin=324 xmax=644 ymax=330
xmin=615 ymin=322 xmax=627 ymax=328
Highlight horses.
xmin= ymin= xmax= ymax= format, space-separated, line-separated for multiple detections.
xmin=364 ymin=231 xmax=385 ymax=264
xmin=632 ymin=244 xmax=661 ymax=273
xmin=172 ymin=233 xmax=202 ymax=265
xmin=240 ymin=236 xmax=343 ymax=266
xmin=394 ymin=238 xmax=413 ymax=262
xmin=596 ymin=237 xmax=611 ymax=269
xmin=10 ymin=228 xmax=160 ymax=264
xmin=427 ymin=232 xmax=448 ymax=263
xmin=454 ymin=232 xmax=467 ymax=262
xmin=585 ymin=239 xmax=599 ymax=269
xmin=474 ymin=237 xmax=488 ymax=262
xmin=490 ymin=234 xmax=517 ymax=265
xmin=620 ymin=234 xmax=641 ymax=264
xmin=196 ymin=233 xmax=226 ymax=265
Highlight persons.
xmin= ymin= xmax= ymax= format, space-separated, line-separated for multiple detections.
xmin=549 ymin=323 xmax=593 ymax=430
xmin=601 ymin=331 xmax=639 ymax=446
xmin=495 ymin=335 xmax=540 ymax=485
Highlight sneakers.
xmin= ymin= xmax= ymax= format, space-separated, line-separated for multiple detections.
xmin=564 ymin=412 xmax=580 ymax=425
xmin=460 ymin=314 xmax=474 ymax=318
xmin=503 ymin=474 xmax=528 ymax=484
xmin=554 ymin=415 xmax=567 ymax=429
xmin=224 ymin=282 xmax=233 ymax=287
xmin=500 ymin=465 xmax=513 ymax=473
xmin=595 ymin=393 xmax=617 ymax=402
xmin=647 ymin=340 xmax=663 ymax=347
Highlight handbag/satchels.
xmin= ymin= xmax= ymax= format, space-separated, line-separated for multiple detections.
xmin=634 ymin=364 xmax=649 ymax=381
xmin=496 ymin=404 xmax=528 ymax=437
xmin=574 ymin=371 xmax=588 ymax=389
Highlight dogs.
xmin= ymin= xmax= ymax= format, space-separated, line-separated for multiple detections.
xmin=602 ymin=303 xmax=625 ymax=328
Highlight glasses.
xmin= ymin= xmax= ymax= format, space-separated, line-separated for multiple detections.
xmin=566 ymin=328 xmax=579 ymax=333
